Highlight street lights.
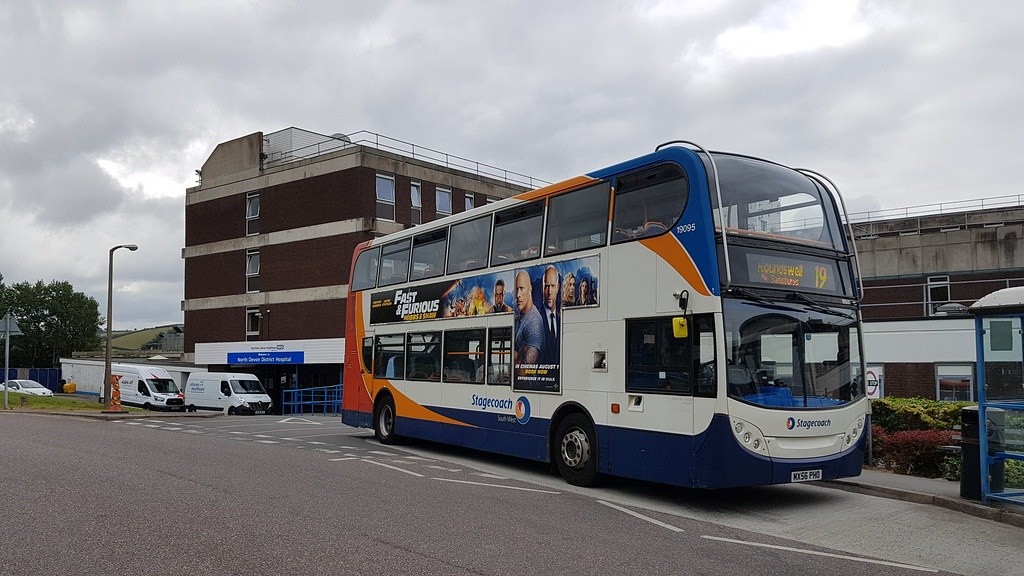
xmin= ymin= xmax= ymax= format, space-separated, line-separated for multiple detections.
xmin=100 ymin=244 xmax=138 ymax=407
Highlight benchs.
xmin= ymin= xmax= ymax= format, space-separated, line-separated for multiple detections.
xmin=937 ymin=425 xmax=1024 ymax=454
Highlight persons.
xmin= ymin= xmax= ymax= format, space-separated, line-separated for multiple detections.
xmin=486 ymin=277 xmax=513 ymax=313
xmin=561 ymin=272 xmax=576 ymax=307
xmin=538 ymin=265 xmax=560 ymax=365
xmin=515 ymin=270 xmax=546 ymax=390
xmin=576 ymin=277 xmax=591 ymax=305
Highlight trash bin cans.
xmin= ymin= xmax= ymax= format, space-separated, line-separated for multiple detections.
xmin=960 ymin=406 xmax=1007 ymax=500
xmin=63 ymin=384 xmax=76 ymax=394
xmin=58 ymin=379 xmax=66 ymax=393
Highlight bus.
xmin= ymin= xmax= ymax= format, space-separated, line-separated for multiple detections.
xmin=339 ymin=139 xmax=871 ymax=491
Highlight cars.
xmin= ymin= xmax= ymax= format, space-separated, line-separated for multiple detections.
xmin=0 ymin=380 xmax=54 ymax=398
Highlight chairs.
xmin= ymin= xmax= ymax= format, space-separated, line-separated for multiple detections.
xmin=612 ymin=223 xmax=672 ymax=243
xmin=381 ymin=353 xmax=511 ymax=389
xmin=361 ymin=236 xmax=560 ymax=286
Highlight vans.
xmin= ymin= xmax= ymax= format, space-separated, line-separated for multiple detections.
xmin=100 ymin=364 xmax=188 ymax=411
xmin=184 ymin=372 xmax=274 ymax=416
